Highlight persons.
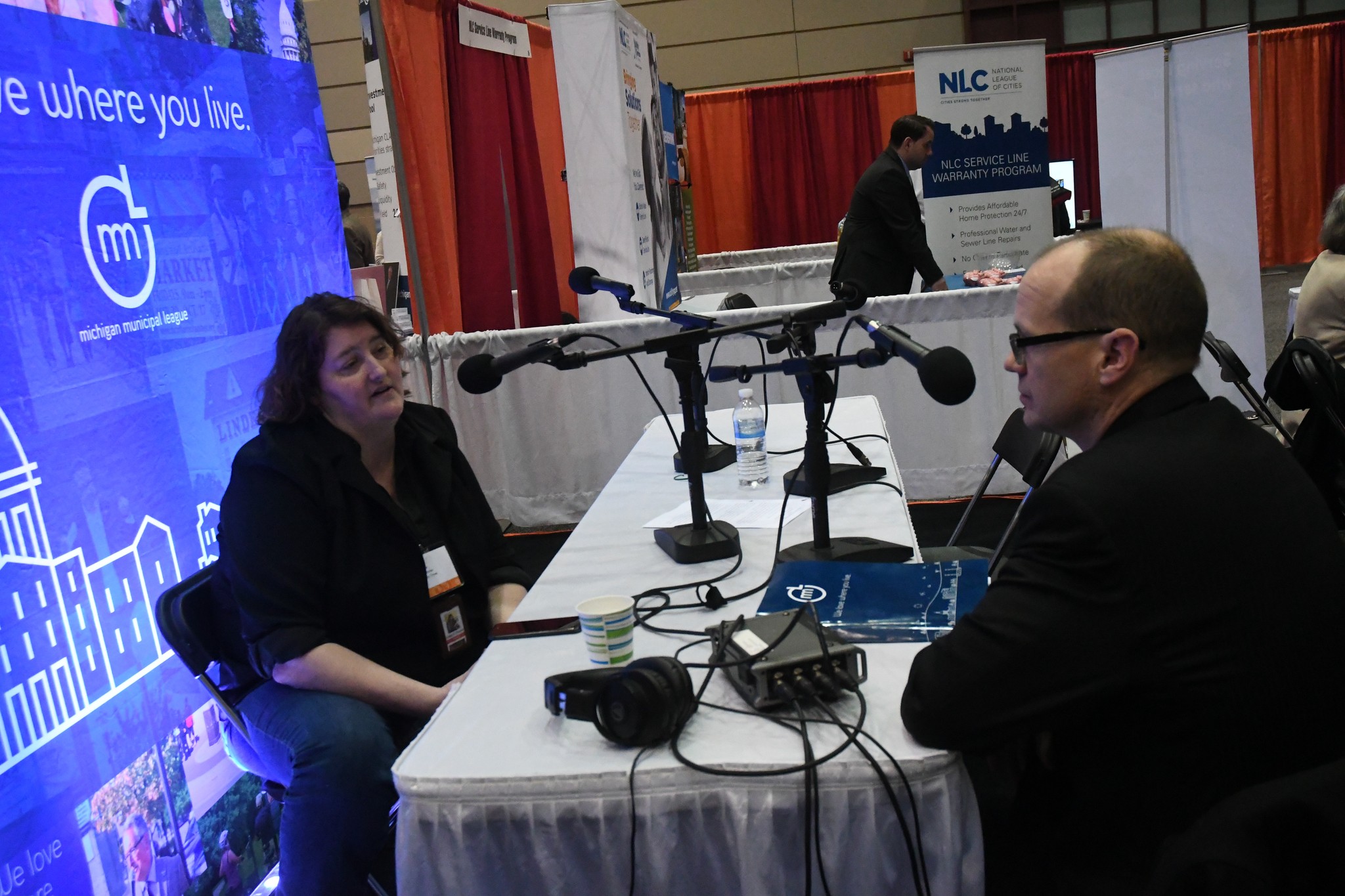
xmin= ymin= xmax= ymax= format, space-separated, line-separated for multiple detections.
xmin=901 ymin=224 xmax=1345 ymax=896
xmin=447 ymin=614 xmax=460 ymax=633
xmin=828 ymin=114 xmax=949 ymax=300
xmin=23 ymin=268 xmax=94 ymax=371
xmin=210 ymin=163 xmax=345 ymax=333
xmin=338 ymin=180 xmax=375 ymax=270
xmin=642 ymin=29 xmax=672 ymax=309
xmin=219 ymin=830 xmax=249 ymax=896
xmin=255 ymin=791 xmax=282 ymax=871
xmin=1275 ymin=184 xmax=1345 ymax=449
xmin=217 ymin=289 xmax=530 ymax=896
xmin=124 ymin=813 xmax=189 ymax=896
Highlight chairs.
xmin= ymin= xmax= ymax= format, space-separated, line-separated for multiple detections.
xmin=919 ymin=407 xmax=1064 ymax=580
xmin=1202 ymin=331 xmax=1294 ymax=446
xmin=155 ymin=560 xmax=400 ymax=896
xmin=1292 ymin=350 xmax=1345 ymax=439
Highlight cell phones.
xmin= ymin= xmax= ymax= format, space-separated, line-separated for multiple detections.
xmin=488 ymin=614 xmax=583 ymax=638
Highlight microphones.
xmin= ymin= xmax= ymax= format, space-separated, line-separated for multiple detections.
xmin=568 ymin=267 xmax=635 ymax=296
xmin=830 ymin=281 xmax=867 ymax=311
xmin=854 ymin=314 xmax=975 ymax=406
xmin=457 ymin=333 xmax=582 ymax=394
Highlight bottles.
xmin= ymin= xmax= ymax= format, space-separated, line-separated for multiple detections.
xmin=732 ymin=389 xmax=770 ymax=487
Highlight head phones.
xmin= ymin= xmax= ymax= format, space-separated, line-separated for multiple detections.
xmin=543 ymin=656 xmax=695 ymax=747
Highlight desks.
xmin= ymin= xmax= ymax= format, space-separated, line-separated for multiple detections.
xmin=391 ymin=394 xmax=982 ymax=896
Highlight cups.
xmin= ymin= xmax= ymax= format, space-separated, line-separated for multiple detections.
xmin=1082 ymin=209 xmax=1090 ymax=221
xmin=577 ymin=595 xmax=635 ymax=668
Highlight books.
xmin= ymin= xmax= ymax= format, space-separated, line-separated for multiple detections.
xmin=756 ymin=560 xmax=989 ymax=643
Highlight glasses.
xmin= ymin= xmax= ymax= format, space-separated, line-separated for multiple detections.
xmin=1009 ymin=326 xmax=1148 ymax=364
